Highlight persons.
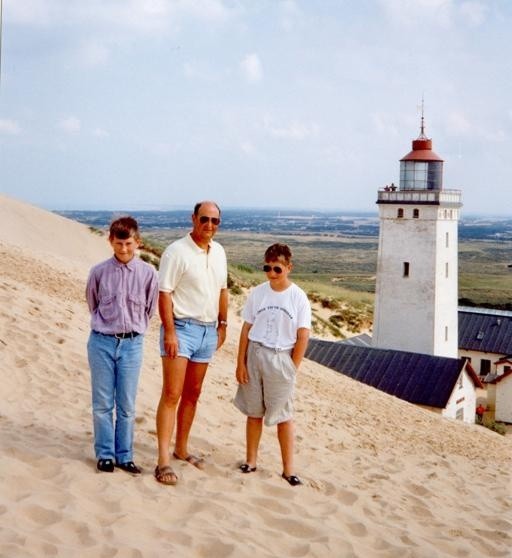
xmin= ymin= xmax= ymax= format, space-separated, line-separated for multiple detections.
xmin=82 ymin=213 xmax=161 ymax=474
xmin=475 ymin=404 xmax=484 ymax=421
xmin=232 ymin=242 xmax=317 ymax=486
xmin=384 ymin=185 xmax=390 ymax=191
xmin=389 ymin=182 xmax=398 ymax=191
xmin=152 ymin=199 xmax=233 ymax=486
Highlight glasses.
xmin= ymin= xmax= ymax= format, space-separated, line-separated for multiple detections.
xmin=262 ymin=265 xmax=283 ymax=273
xmin=200 ymin=216 xmax=220 ymax=226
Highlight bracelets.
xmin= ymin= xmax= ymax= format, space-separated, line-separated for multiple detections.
xmin=219 ymin=320 xmax=228 ymax=327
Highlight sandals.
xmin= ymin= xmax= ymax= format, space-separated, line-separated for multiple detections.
xmin=173 ymin=452 xmax=207 ymax=471
xmin=154 ymin=465 xmax=178 ymax=486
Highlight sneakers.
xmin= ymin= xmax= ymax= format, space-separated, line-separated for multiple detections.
xmin=116 ymin=461 xmax=142 ymax=476
xmin=97 ymin=458 xmax=115 ymax=473
xmin=282 ymin=472 xmax=302 ymax=487
xmin=235 ymin=463 xmax=256 ymax=475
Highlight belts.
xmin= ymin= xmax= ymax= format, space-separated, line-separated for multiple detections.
xmin=93 ymin=328 xmax=141 ymax=340
